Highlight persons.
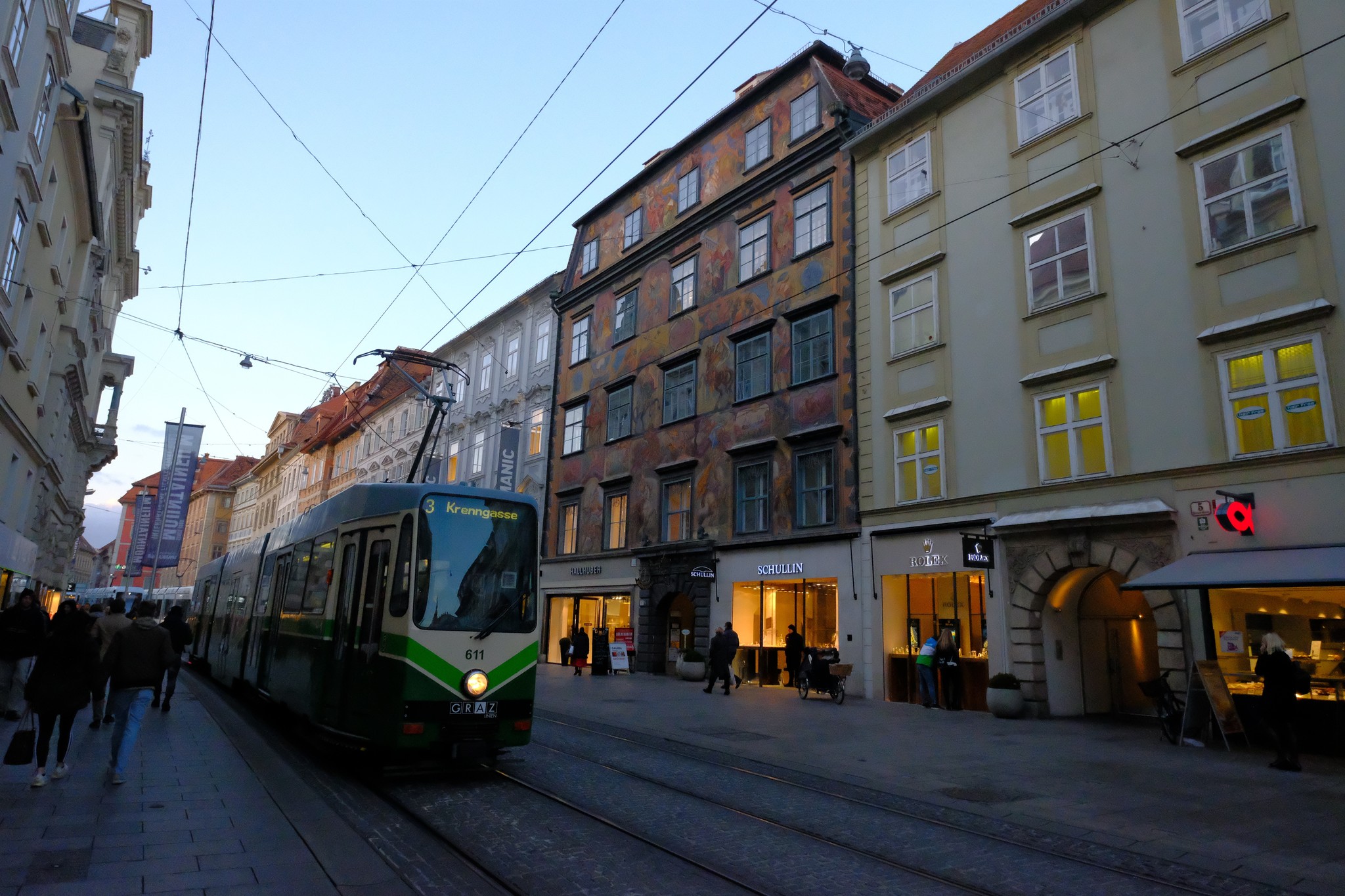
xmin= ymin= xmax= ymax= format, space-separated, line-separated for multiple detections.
xmin=720 ymin=621 xmax=742 ymax=688
xmin=784 ymin=625 xmax=804 ymax=687
xmin=1 ymin=583 xmax=198 ymax=786
xmin=572 ymin=627 xmax=590 ymax=675
xmin=915 ymin=633 xmax=945 ymax=710
xmin=702 ymin=627 xmax=732 ymax=695
xmin=936 ymin=628 xmax=962 ymax=711
xmin=1254 ymin=632 xmax=1301 ymax=770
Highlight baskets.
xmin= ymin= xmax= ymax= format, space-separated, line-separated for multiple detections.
xmin=1139 ymin=678 xmax=1168 ymax=698
xmin=829 ymin=662 xmax=854 ymax=676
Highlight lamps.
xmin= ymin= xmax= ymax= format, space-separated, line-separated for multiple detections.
xmin=198 ymin=456 xmax=207 ymax=466
xmin=239 ymin=354 xmax=253 ymax=370
xmin=840 ymin=43 xmax=871 ymax=83
xmin=301 ymin=468 xmax=309 ymax=475
xmin=415 ymin=391 xmax=426 ymax=405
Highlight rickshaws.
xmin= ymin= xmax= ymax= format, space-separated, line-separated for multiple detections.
xmin=797 ymin=646 xmax=847 ymax=705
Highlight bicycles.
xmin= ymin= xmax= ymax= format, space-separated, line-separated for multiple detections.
xmin=1141 ymin=669 xmax=1186 ymax=746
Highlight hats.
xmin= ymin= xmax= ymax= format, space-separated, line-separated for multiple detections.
xmin=19 ymin=588 xmax=35 ymax=602
xmin=788 ymin=624 xmax=795 ymax=631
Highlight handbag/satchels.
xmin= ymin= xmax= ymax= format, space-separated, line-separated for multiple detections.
xmin=1292 ymin=663 xmax=1311 ymax=695
xmin=565 ymin=642 xmax=574 ymax=658
xmin=568 ymin=642 xmax=574 ymax=655
xmin=3 ymin=704 xmax=36 ymax=766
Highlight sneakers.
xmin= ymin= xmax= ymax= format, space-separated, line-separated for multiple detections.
xmin=53 ymin=763 xmax=69 ymax=779
xmin=31 ymin=772 xmax=49 ymax=786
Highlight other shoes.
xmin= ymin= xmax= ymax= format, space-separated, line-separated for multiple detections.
xmin=112 ymin=769 xmax=130 ymax=784
xmin=103 ymin=715 xmax=115 ymax=723
xmin=702 ymin=679 xmax=742 ymax=695
xmin=161 ymin=697 xmax=170 ymax=711
xmin=784 ymin=682 xmax=794 ymax=687
xmin=1267 ymin=753 xmax=1291 ymax=771
xmin=922 ymin=701 xmax=964 ymax=711
xmin=151 ymin=696 xmax=160 ymax=707
xmin=109 ymin=767 xmax=114 ymax=776
xmin=1288 ymin=752 xmax=1302 ymax=771
xmin=88 ymin=720 xmax=100 ymax=728
xmin=1 ymin=706 xmax=20 ymax=721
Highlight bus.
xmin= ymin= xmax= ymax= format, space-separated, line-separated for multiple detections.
xmin=143 ymin=585 xmax=194 ymax=624
xmin=83 ymin=586 xmax=145 ymax=616
xmin=184 ymin=481 xmax=543 ymax=758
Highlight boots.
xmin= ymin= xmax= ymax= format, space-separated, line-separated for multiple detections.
xmin=574 ymin=667 xmax=578 ymax=675
xmin=578 ymin=670 xmax=582 ymax=676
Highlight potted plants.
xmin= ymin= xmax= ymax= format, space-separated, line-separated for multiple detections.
xmin=986 ymin=673 xmax=1027 ymax=718
xmin=673 ymin=647 xmax=693 ymax=676
xmin=681 ymin=651 xmax=706 ymax=680
xmin=559 ymin=638 xmax=571 ymax=666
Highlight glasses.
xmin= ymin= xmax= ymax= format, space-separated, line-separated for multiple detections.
xmin=723 ymin=626 xmax=725 ymax=628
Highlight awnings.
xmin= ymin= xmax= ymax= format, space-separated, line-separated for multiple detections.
xmin=1118 ymin=545 xmax=1345 ymax=593
